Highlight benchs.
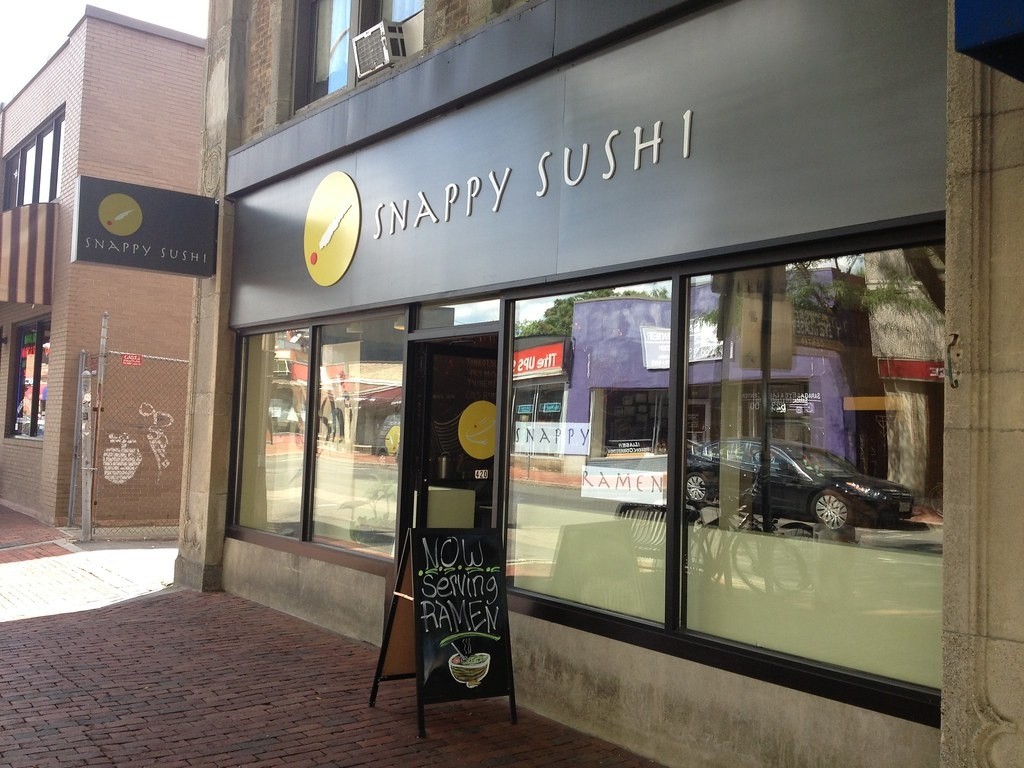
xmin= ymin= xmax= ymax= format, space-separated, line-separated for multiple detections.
xmin=616 ymin=503 xmax=700 ymax=561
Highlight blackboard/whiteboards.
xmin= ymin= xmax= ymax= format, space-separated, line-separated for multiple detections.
xmin=375 ymin=527 xmax=516 ymax=706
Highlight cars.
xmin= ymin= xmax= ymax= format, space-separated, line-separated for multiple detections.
xmin=684 ymin=439 xmax=916 ymax=527
